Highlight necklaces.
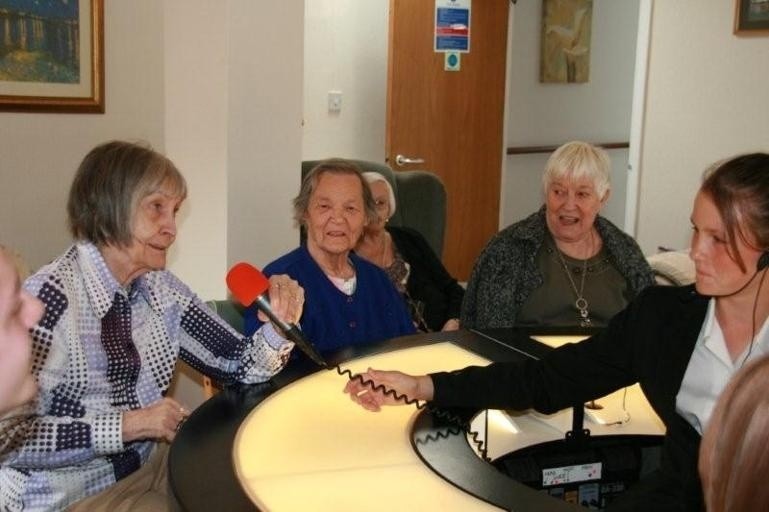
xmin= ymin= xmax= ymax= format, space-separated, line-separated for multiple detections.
xmin=553 ymin=234 xmax=597 ymax=327
xmin=358 ymin=231 xmax=393 ymax=272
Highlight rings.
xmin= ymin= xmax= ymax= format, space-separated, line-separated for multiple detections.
xmin=176 ymin=415 xmax=187 ymax=424
xmin=179 ymin=405 xmax=186 ymax=415
xmin=269 ymin=283 xmax=279 ymax=289
xmin=281 ymin=284 xmax=289 ymax=289
xmin=171 ymin=421 xmax=181 ymax=432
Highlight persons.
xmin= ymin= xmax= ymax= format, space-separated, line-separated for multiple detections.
xmin=461 ymin=140 xmax=660 ymax=329
xmin=0 ymin=244 xmax=46 ymax=416
xmin=245 ymin=156 xmax=419 ymax=358
xmin=697 ymin=352 xmax=769 ymax=512
xmin=340 ymin=152 xmax=769 ymax=511
xmin=357 ymin=168 xmax=466 ymax=334
xmin=1 ymin=140 xmax=305 ymax=512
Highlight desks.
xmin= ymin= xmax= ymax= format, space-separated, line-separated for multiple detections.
xmin=168 ymin=324 xmax=667 ymax=512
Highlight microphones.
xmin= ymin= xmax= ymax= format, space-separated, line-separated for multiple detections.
xmin=223 ymin=261 xmax=327 ymax=366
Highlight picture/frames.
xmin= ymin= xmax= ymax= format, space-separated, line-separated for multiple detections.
xmin=733 ymin=0 xmax=769 ymax=38
xmin=1 ymin=1 xmax=107 ymax=115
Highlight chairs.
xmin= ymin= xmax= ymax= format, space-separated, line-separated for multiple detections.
xmin=298 ymin=160 xmax=447 ymax=264
xmin=205 ymin=301 xmax=249 ymax=400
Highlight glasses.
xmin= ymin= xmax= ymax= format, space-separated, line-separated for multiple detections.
xmin=374 ymin=199 xmax=391 ymax=211
